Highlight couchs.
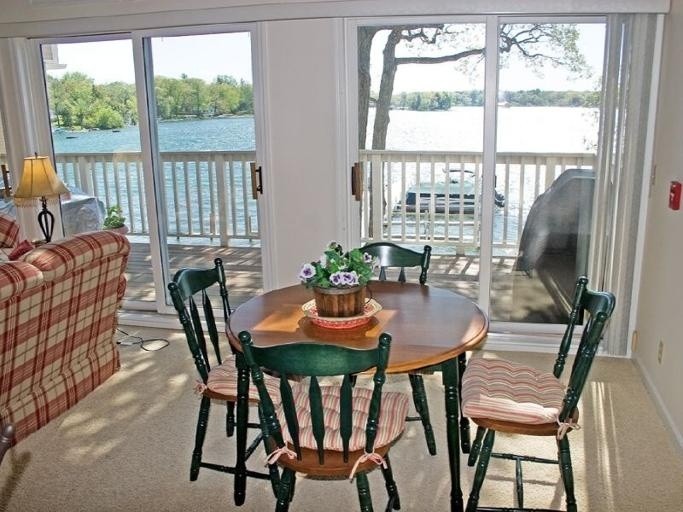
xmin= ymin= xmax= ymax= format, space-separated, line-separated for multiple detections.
xmin=0 ymin=230 xmax=131 ymax=450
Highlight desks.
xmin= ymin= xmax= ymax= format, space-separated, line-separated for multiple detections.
xmin=0 ymin=194 xmax=106 ymax=237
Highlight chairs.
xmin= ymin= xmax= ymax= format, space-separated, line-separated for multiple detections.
xmin=0 ymin=213 xmax=20 ymax=248
xmin=458 ymin=275 xmax=616 ymax=512
xmin=168 ymin=257 xmax=300 ymax=500
xmin=337 ymin=242 xmax=439 ymax=456
xmin=237 ymin=329 xmax=401 ymax=512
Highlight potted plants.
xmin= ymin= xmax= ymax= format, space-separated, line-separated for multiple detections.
xmin=102 ymin=204 xmax=129 ymax=235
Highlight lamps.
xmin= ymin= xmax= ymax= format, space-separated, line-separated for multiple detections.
xmin=13 ymin=152 xmax=71 ymax=242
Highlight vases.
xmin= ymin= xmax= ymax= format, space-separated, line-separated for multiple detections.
xmin=310 ymin=285 xmax=373 ymax=317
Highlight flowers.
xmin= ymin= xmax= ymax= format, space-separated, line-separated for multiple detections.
xmin=296 ymin=239 xmax=381 ymax=290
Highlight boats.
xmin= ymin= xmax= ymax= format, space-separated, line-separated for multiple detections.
xmin=395 ymin=167 xmax=504 ymax=213
